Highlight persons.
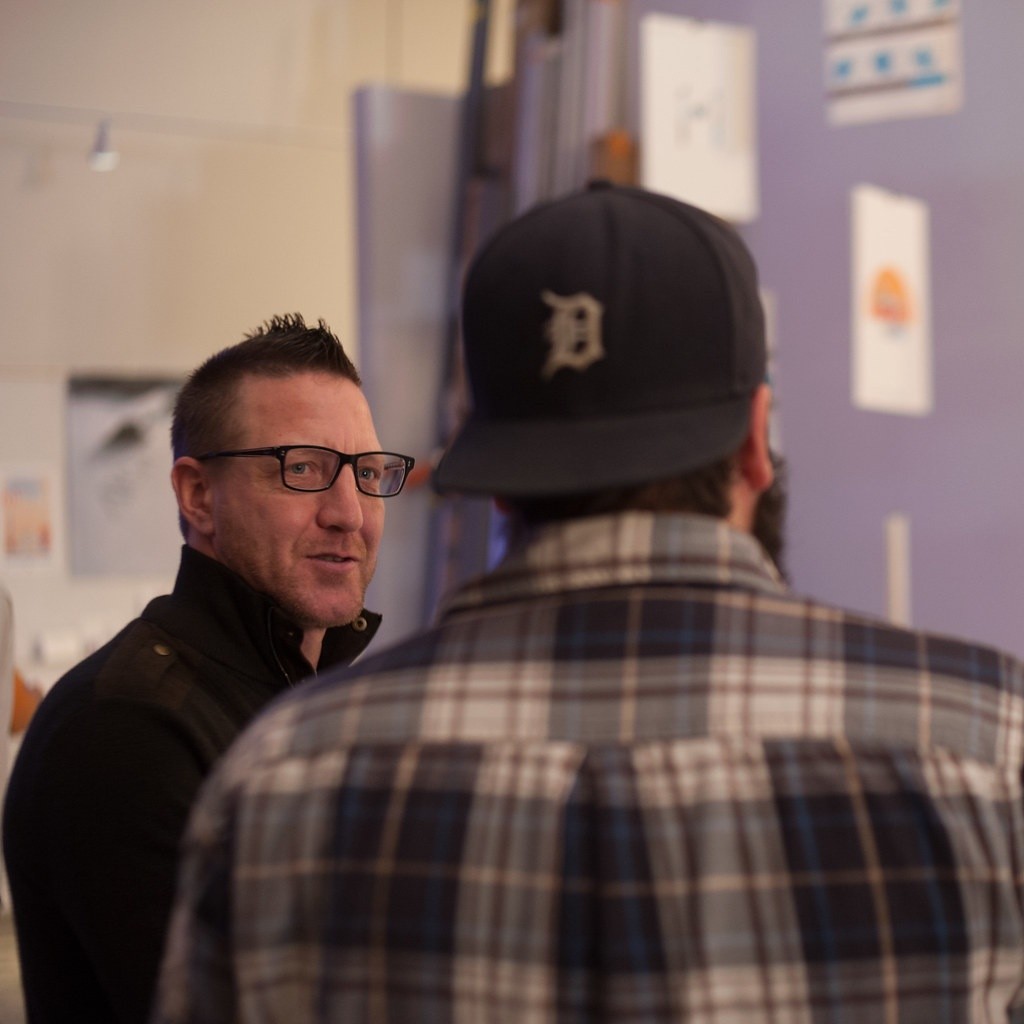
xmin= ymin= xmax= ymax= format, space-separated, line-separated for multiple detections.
xmin=0 ymin=312 xmax=414 ymax=1023
xmin=166 ymin=178 xmax=1024 ymax=1023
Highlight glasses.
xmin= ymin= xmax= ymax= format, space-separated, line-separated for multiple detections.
xmin=194 ymin=444 xmax=416 ymax=498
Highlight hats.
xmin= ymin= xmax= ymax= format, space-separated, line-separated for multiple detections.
xmin=426 ymin=178 xmax=765 ymax=496
